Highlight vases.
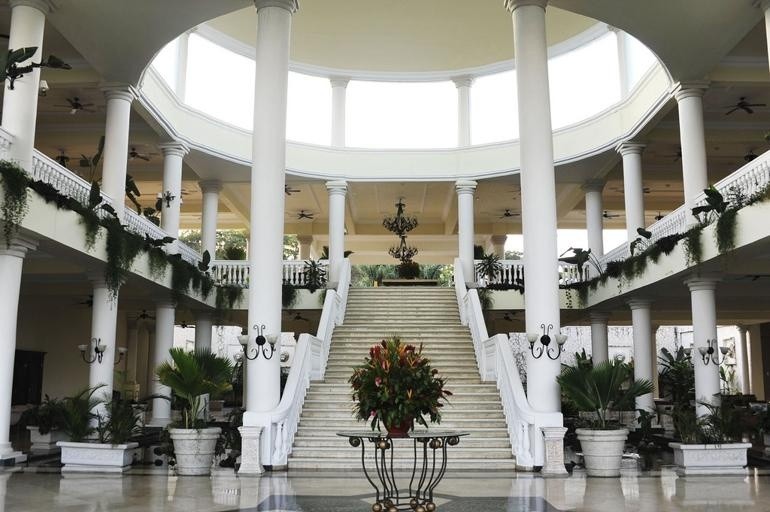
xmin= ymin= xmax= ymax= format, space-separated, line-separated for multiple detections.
xmin=379 ymin=411 xmax=414 ymax=437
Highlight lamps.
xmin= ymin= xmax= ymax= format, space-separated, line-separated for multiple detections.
xmin=526 ymin=323 xmax=567 ymax=361
xmin=388 ymin=236 xmax=418 ymax=261
xmin=683 ymin=347 xmax=695 ymax=367
xmin=114 ymin=346 xmax=126 ymax=367
xmin=697 ymin=338 xmax=730 ymax=365
xmin=379 ymin=197 xmax=418 ymax=235
xmin=38 ymin=79 xmax=49 ymax=97
xmin=236 ymin=325 xmax=277 ymax=360
xmin=77 ymin=338 xmax=106 ymax=364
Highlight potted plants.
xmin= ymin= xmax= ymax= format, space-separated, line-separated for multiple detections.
xmin=54 ymin=386 xmax=139 ymax=474
xmin=661 ymin=359 xmax=754 ymax=475
xmin=155 ymin=348 xmax=230 ymax=475
xmin=24 ymin=397 xmax=82 ymax=451
xmin=556 ymin=361 xmax=654 ymax=479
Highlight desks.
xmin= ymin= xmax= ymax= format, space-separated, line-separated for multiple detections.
xmin=336 ymin=429 xmax=469 ymax=511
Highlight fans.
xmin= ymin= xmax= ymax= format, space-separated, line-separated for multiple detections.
xmin=72 ymin=295 xmax=93 ymax=308
xmin=493 ymin=312 xmax=521 ymax=323
xmin=296 ymin=209 xmax=315 ymax=221
xmin=722 ymin=97 xmax=768 ymax=117
xmin=494 ymin=209 xmax=521 ymax=219
xmin=131 ymin=310 xmax=156 ymax=321
xmin=175 ymin=320 xmax=196 ymax=329
xmin=601 ymin=209 xmax=622 ymax=221
xmin=128 ymin=145 xmax=150 ymax=162
xmin=52 ymin=95 xmax=98 ymax=117
xmin=663 ymin=147 xmax=682 ymax=162
xmin=285 ymin=184 xmax=301 ymax=196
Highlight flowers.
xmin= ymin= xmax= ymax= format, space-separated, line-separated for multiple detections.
xmin=350 ymin=335 xmax=456 ymax=428
